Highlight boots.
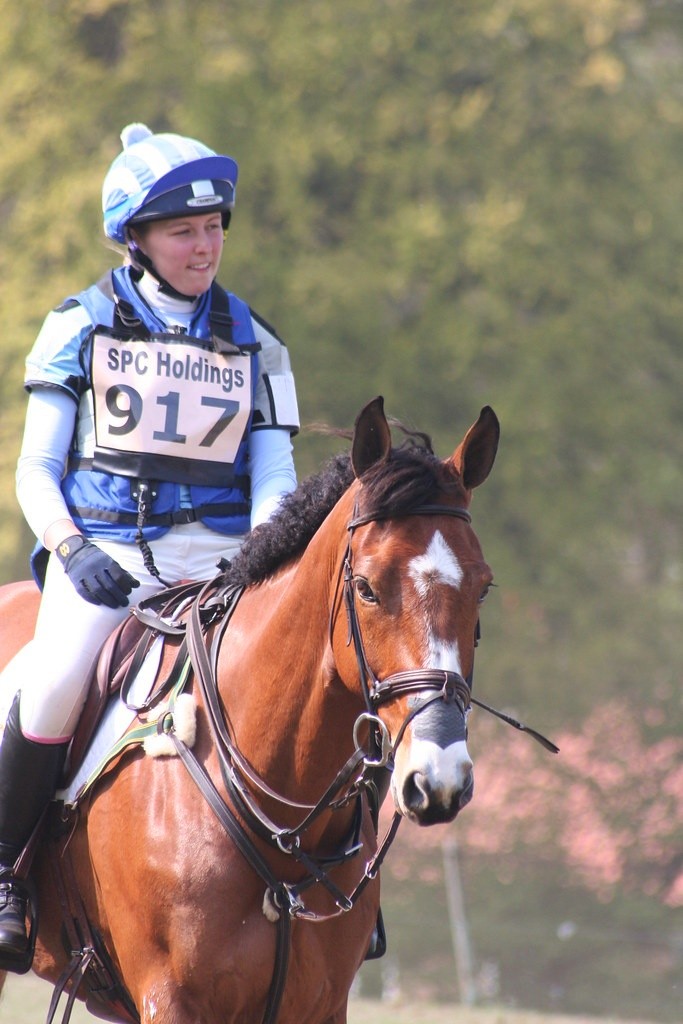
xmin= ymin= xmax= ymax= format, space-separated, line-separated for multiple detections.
xmin=0 ymin=689 xmax=71 ymax=952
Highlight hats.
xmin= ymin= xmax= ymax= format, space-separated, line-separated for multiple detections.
xmin=102 ymin=122 xmax=238 ymax=245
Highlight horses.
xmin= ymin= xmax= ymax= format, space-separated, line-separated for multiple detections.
xmin=0 ymin=395 xmax=500 ymax=1024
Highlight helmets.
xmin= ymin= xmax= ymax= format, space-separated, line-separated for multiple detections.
xmin=125 ymin=181 xmax=233 ymax=230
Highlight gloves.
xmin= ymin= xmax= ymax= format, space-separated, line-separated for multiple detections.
xmin=56 ymin=534 xmax=140 ymax=609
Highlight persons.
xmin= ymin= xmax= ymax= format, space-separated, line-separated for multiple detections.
xmin=0 ymin=123 xmax=382 ymax=974
